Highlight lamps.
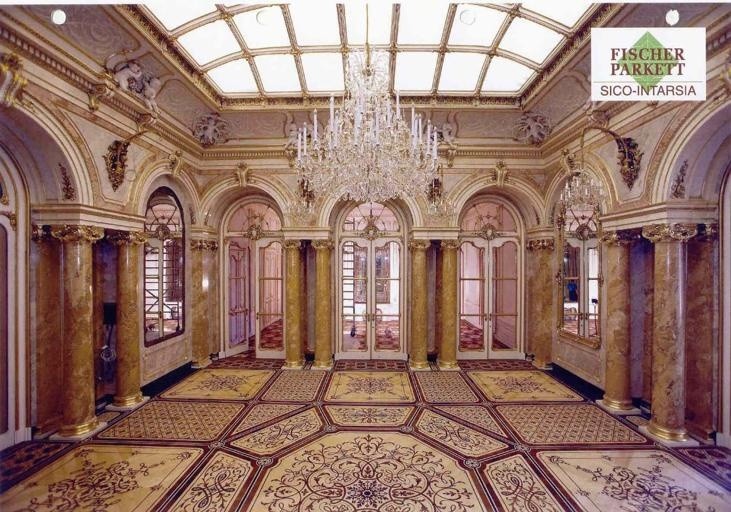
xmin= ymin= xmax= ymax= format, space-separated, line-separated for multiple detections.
xmin=283 ymin=4 xmax=438 ymax=201
xmin=556 ymin=145 xmax=609 ymax=209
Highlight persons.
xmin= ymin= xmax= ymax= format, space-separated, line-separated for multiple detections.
xmin=567 ymin=280 xmax=577 ymax=302
